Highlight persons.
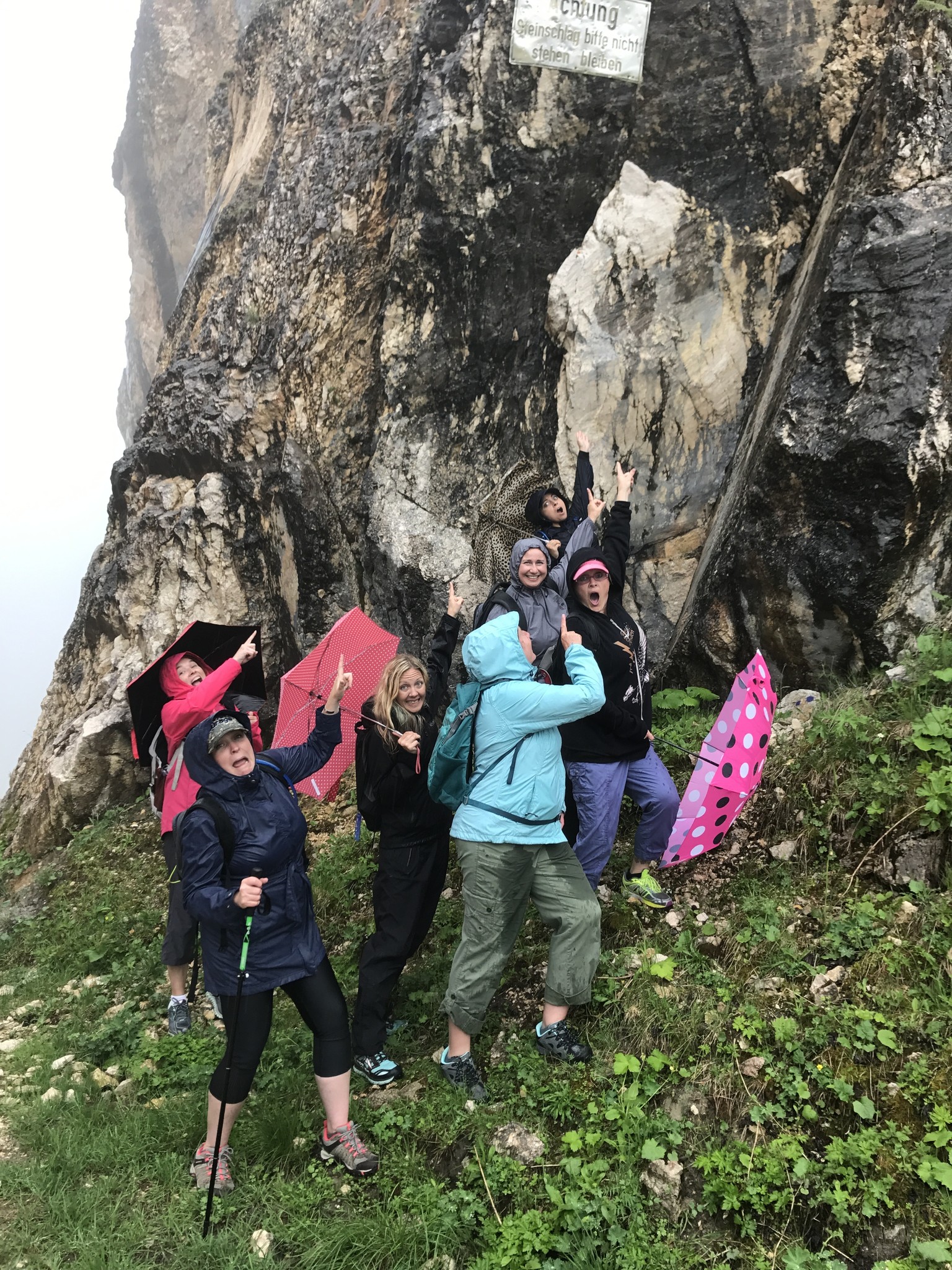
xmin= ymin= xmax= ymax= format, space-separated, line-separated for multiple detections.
xmin=156 ymin=629 xmax=263 ymax=1035
xmin=437 ymin=611 xmax=606 ymax=1108
xmin=172 ymin=652 xmax=379 ymax=1195
xmin=349 ymin=579 xmax=464 ymax=1086
xmin=470 ymin=424 xmax=680 ymax=911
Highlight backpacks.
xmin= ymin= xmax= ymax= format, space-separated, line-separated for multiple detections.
xmin=470 ymin=577 xmax=560 ymax=632
xmin=426 ymin=678 xmax=516 ymax=811
xmin=350 ymin=720 xmax=400 ymax=830
xmin=171 ymin=759 xmax=299 ymax=928
xmin=149 ymin=724 xmax=190 ymax=819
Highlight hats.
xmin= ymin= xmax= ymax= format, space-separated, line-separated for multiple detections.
xmin=572 ymin=559 xmax=609 ymax=580
xmin=206 ymin=715 xmax=248 ymax=753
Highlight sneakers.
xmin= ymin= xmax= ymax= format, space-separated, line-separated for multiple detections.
xmin=320 ymin=1119 xmax=379 ymax=1173
xmin=189 ymin=1142 xmax=234 ymax=1195
xmin=440 ymin=1046 xmax=488 ymax=1103
xmin=166 ymin=996 xmax=193 ymax=1036
xmin=353 ymin=1051 xmax=403 ymax=1084
xmin=206 ymin=991 xmax=223 ymax=1019
xmin=535 ymin=1020 xmax=593 ymax=1062
xmin=620 ymin=868 xmax=673 ymax=908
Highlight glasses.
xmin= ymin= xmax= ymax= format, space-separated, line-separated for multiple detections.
xmin=576 ymin=573 xmax=608 ymax=584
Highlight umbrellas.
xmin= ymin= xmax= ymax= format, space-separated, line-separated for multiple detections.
xmin=652 ymin=645 xmax=778 ymax=870
xmin=468 ymin=457 xmax=563 ymax=585
xmin=125 ymin=619 xmax=269 ymax=770
xmin=270 ymin=604 xmax=422 ymax=801
